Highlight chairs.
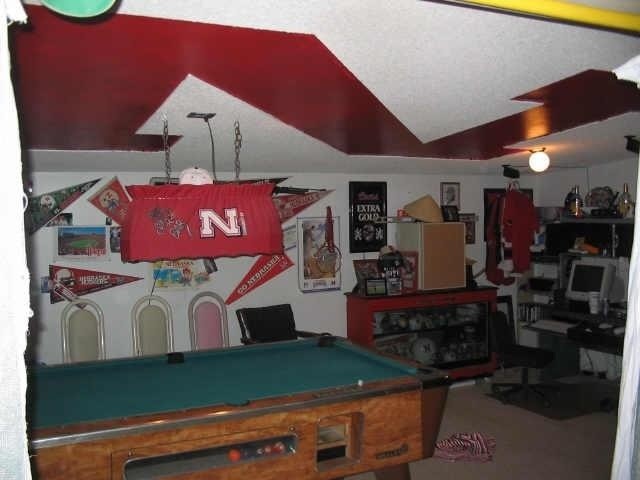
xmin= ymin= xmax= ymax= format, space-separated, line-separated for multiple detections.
xmin=353 ymin=252 xmax=386 ymax=291
xmin=497 ymin=295 xmax=554 ymax=406
xmin=188 ymin=291 xmax=229 ymax=351
xmin=236 ymin=303 xmax=332 ymax=345
xmin=131 ymin=295 xmax=173 ymax=357
xmin=60 ymin=299 xmax=106 ymax=363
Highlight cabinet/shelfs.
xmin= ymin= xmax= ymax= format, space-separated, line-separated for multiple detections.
xmin=545 ymin=223 xmax=633 ymax=299
xmin=343 ymin=286 xmax=497 ymax=379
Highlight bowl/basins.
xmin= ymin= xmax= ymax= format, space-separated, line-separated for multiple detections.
xmin=529 ymin=278 xmax=554 ymax=291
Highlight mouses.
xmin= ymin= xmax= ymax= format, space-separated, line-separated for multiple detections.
xmin=598 ymin=322 xmax=613 ymax=329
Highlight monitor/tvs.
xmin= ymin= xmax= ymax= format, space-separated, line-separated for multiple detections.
xmin=564 ymin=259 xmax=618 ymax=302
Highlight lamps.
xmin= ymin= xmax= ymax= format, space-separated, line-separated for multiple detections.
xmin=529 ymin=148 xmax=550 ymax=172
xmin=122 ymin=119 xmax=284 ymax=262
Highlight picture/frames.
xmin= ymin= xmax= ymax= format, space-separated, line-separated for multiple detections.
xmin=484 ymin=187 xmax=534 ymax=241
xmin=400 ymin=251 xmax=418 ymax=293
xmin=440 ymin=182 xmax=475 ymax=244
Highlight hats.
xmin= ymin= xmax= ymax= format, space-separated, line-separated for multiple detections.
xmin=404 ymin=195 xmax=443 ymax=223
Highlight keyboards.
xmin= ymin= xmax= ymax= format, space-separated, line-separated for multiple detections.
xmin=530 ymin=319 xmax=577 ymax=335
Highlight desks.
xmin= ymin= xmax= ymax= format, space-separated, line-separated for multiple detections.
xmin=28 ymin=336 xmax=451 ymax=480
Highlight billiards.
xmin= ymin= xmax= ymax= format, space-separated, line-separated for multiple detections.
xmin=229 ymin=439 xmax=295 ymax=463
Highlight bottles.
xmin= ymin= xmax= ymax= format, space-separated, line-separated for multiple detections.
xmin=618 ymin=183 xmax=632 ymax=218
xmin=571 ymin=185 xmax=583 ymax=218
xmin=402 ymin=264 xmax=416 ymax=295
xmin=589 ymin=292 xmax=600 ymax=314
xmin=386 ymin=267 xmax=403 ymax=296
